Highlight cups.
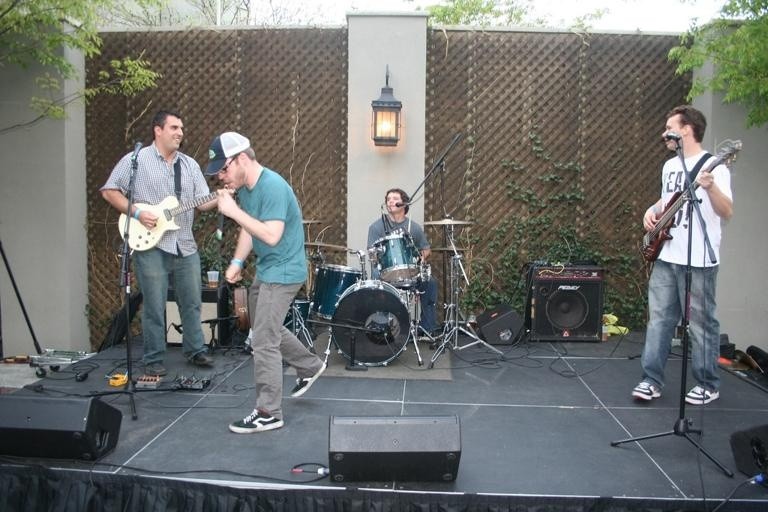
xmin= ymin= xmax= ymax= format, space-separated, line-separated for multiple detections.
xmin=206 ymin=270 xmax=219 ymax=288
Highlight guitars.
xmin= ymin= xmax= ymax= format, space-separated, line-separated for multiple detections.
xmin=642 ymin=141 xmax=743 ymax=260
xmin=118 ymin=189 xmax=236 ymax=252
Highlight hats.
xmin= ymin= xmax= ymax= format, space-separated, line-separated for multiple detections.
xmin=203 ymin=132 xmax=250 ymax=176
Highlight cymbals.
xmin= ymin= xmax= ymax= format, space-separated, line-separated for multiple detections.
xmin=424 ymin=219 xmax=473 ymax=226
xmin=431 ymin=246 xmax=467 ymax=252
xmin=303 ymin=240 xmax=350 ymax=253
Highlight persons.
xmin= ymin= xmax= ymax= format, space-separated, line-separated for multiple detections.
xmin=365 ymin=187 xmax=442 ymax=342
xmin=205 ymin=129 xmax=329 ymax=436
xmin=632 ymin=105 xmax=735 ymax=405
xmin=101 ymin=108 xmax=236 ymax=379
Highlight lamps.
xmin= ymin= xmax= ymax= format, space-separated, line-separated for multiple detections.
xmin=370 ymin=65 xmax=403 ymax=147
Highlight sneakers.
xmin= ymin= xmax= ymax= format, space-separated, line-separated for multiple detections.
xmin=140 ymin=363 xmax=166 ymax=376
xmin=228 ymin=408 xmax=283 ymax=434
xmin=189 ymin=351 xmax=213 ymax=365
xmin=684 ymin=386 xmax=718 ymax=406
xmin=631 ymin=382 xmax=661 ymax=400
xmin=290 ymin=363 xmax=326 ymax=398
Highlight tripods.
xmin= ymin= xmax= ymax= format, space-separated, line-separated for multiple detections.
xmin=405 ymin=230 xmax=507 ymax=369
xmin=611 ymin=144 xmax=735 ymax=477
xmin=284 ymin=300 xmax=317 ymax=354
xmin=85 ymin=170 xmax=179 ymax=420
xmin=180 ymin=315 xmax=242 ymax=356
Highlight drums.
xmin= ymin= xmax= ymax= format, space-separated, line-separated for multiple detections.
xmin=373 ymin=233 xmax=421 ymax=286
xmin=283 ymin=299 xmax=309 ymax=331
xmin=330 ymin=280 xmax=412 ymax=368
xmin=308 ymin=263 xmax=368 ymax=320
xmin=398 ymin=288 xmax=422 ymax=322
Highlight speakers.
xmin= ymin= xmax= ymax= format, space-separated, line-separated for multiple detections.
xmin=526 ymin=264 xmax=603 ymax=342
xmin=476 ymin=304 xmax=525 ymax=345
xmin=328 ymin=415 xmax=461 ymax=483
xmin=730 ymin=424 xmax=768 ymax=488
xmin=0 ymin=393 xmax=122 ymax=460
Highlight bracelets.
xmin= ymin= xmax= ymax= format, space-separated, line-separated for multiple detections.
xmin=230 ymin=256 xmax=244 ymax=270
xmin=649 ymin=205 xmax=659 ymax=215
xmin=132 ymin=208 xmax=142 ymax=219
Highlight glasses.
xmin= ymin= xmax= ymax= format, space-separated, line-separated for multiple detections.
xmin=219 ymin=158 xmax=235 ymax=173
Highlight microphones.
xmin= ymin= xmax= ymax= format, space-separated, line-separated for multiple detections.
xmin=131 ymin=142 xmax=144 ymax=164
xmin=666 ymin=133 xmax=681 ymax=139
xmin=173 ymin=323 xmax=183 ymax=335
xmin=216 ymin=188 xmax=224 ymax=239
xmin=396 ymin=203 xmax=408 ymax=207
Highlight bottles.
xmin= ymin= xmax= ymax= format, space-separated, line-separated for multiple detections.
xmin=602 ymin=324 xmax=607 ymax=341
